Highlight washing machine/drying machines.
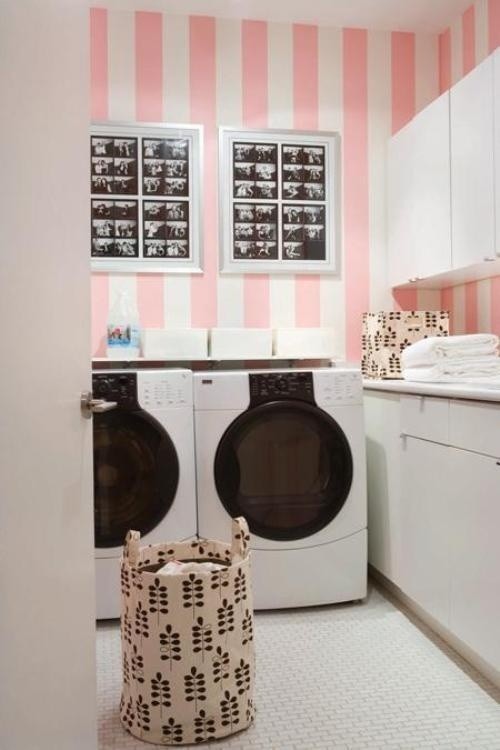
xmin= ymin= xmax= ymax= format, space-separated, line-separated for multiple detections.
xmin=193 ymin=368 xmax=368 ymax=610
xmin=92 ymin=367 xmax=198 ymax=621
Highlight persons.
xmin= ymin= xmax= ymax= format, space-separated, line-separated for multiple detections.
xmin=92 ymin=138 xmax=190 ymax=258
xmin=232 ymin=144 xmax=326 ymax=261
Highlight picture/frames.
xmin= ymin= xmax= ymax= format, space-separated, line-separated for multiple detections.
xmin=89 ymin=120 xmax=206 ymax=274
xmin=217 ymin=124 xmax=340 ymax=276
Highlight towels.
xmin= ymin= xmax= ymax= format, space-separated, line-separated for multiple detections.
xmin=399 ymin=333 xmax=500 ymax=380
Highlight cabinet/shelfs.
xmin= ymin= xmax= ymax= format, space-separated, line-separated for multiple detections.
xmin=364 ymin=381 xmax=499 ymax=690
xmin=390 ymin=47 xmax=500 ymax=290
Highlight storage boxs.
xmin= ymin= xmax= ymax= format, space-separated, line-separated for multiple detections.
xmin=362 ymin=309 xmax=449 ymax=381
